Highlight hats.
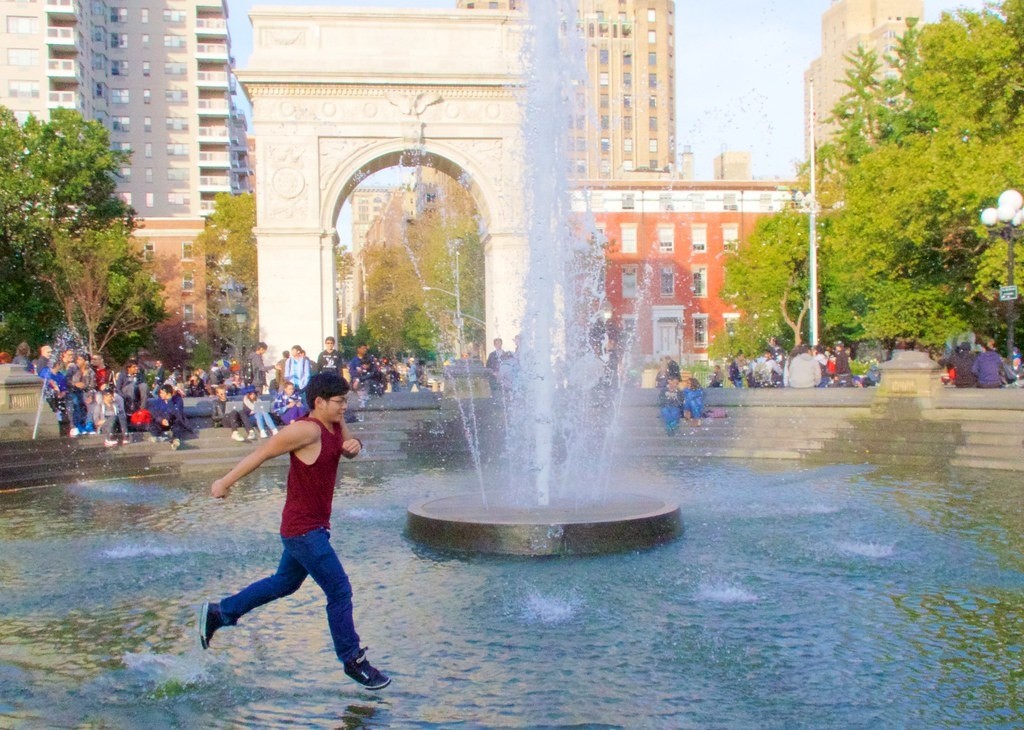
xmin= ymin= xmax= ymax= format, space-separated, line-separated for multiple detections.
xmin=961 ymin=342 xmax=971 ymax=349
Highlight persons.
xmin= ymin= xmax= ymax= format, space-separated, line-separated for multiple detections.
xmin=656 ymin=335 xmax=1024 ymax=437
xmin=0 ymin=326 xmax=520 ymax=452
xmin=201 ymin=371 xmax=391 ymax=691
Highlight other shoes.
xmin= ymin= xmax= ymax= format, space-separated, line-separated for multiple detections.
xmin=667 ymin=422 xmax=678 ymax=435
xmin=78 ymin=424 xmax=88 ymax=436
xmin=86 ymin=423 xmax=96 ymax=436
xmin=123 ymin=435 xmax=130 ymax=444
xmin=105 ymin=439 xmax=118 ymax=444
xmin=171 ymin=439 xmax=180 ymax=450
xmin=151 ymin=436 xmax=159 ymax=444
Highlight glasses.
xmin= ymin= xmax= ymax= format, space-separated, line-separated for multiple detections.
xmin=324 ymin=396 xmax=351 ymax=405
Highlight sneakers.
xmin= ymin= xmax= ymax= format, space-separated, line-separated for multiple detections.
xmin=261 ymin=428 xmax=268 ymax=440
xmin=231 ymin=431 xmax=245 ymax=442
xmin=272 ymin=428 xmax=279 ymax=436
xmin=343 ymin=646 xmax=390 ymax=690
xmin=199 ymin=602 xmax=224 ymax=649
xmin=248 ymin=431 xmax=256 ymax=441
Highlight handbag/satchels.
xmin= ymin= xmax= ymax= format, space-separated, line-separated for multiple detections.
xmin=1001 ymin=365 xmax=1018 ymax=383
xmin=705 ymin=408 xmax=726 ymax=418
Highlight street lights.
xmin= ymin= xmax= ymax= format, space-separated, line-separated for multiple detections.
xmin=979 ymin=189 xmax=1024 ymax=377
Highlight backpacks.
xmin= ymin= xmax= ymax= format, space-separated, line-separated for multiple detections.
xmin=245 ymin=356 xmax=259 ymax=383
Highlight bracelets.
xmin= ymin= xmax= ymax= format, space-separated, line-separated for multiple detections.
xmin=353 ymin=437 xmax=363 ymax=449
